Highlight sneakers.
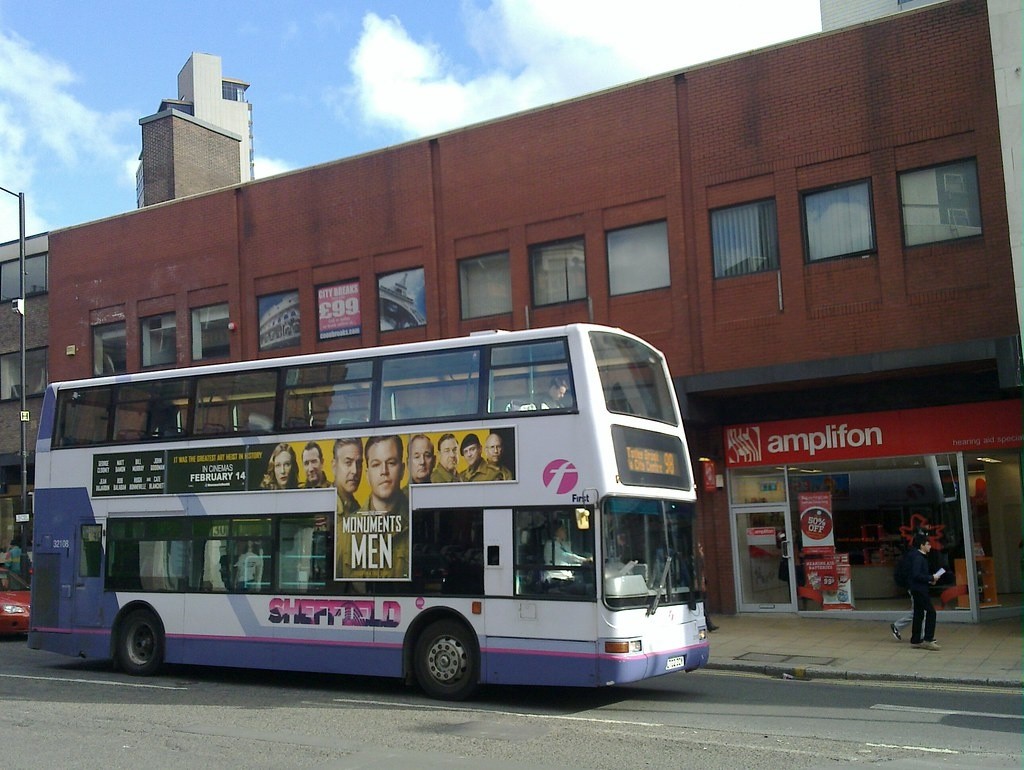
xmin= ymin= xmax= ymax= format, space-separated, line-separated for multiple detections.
xmin=888 ymin=622 xmax=900 ymax=642
xmin=912 ymin=639 xmax=939 ymax=651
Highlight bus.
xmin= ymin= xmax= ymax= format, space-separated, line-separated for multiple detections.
xmin=28 ymin=323 xmax=710 ymax=702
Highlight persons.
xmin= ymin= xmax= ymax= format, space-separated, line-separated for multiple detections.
xmin=335 ymin=436 xmax=410 ymax=579
xmin=777 ymin=529 xmax=810 ymax=610
xmin=485 ymin=434 xmax=514 ymax=481
xmin=301 ymin=441 xmax=332 ymax=488
xmin=544 ymin=518 xmax=593 ymax=594
xmin=697 ymin=542 xmax=719 ymax=632
xmin=400 ymin=435 xmax=436 ymax=498
xmin=458 ymin=433 xmax=487 ymax=482
xmin=231 ymin=540 xmax=263 ymax=592
xmin=430 ymin=433 xmax=460 ymax=483
xmin=536 ymin=376 xmax=568 ymax=411
xmin=0 ymin=540 xmax=33 ymax=590
xmin=329 ymin=438 xmax=363 ymax=523
xmin=890 ymin=536 xmax=943 ymax=652
xmin=263 ymin=444 xmax=300 ymax=490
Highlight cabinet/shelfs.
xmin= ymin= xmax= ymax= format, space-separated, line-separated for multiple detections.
xmin=954 ymin=557 xmax=1002 ymax=611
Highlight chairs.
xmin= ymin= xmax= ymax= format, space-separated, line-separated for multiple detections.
xmin=116 ymin=401 xmax=536 ymax=443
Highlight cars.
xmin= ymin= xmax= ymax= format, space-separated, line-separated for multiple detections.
xmin=411 ymin=544 xmax=483 ymax=564
xmin=381 ymin=554 xmax=451 ymax=594
xmin=0 ymin=568 xmax=31 ymax=637
